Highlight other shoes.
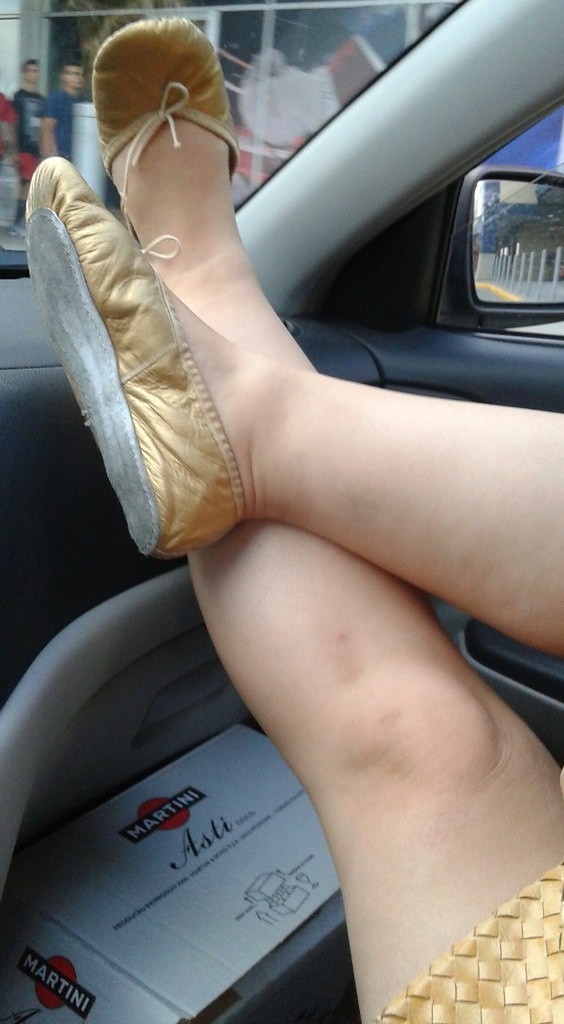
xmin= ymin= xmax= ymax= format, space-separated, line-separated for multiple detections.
xmin=93 ymin=15 xmax=240 ymax=238
xmin=25 ymin=157 xmax=245 ymax=559
xmin=7 ymin=226 xmax=26 ymax=238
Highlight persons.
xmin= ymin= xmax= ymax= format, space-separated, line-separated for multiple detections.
xmin=25 ymin=18 xmax=564 ymax=1024
xmin=0 ymin=59 xmax=85 ymax=238
